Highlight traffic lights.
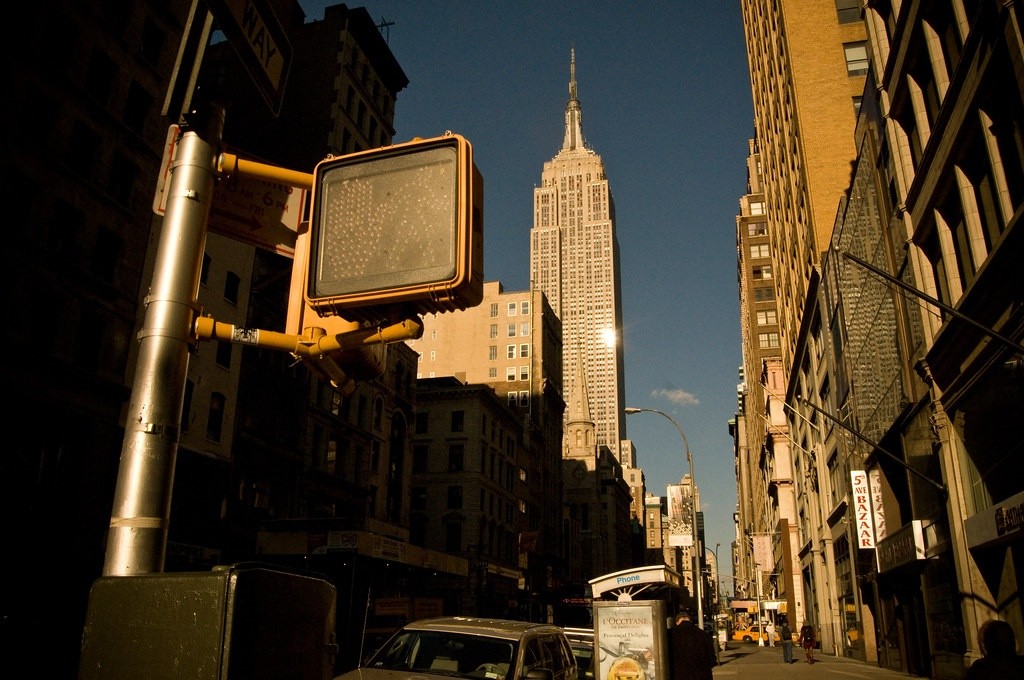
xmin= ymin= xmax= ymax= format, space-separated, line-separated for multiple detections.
xmin=303 ymin=129 xmax=484 ymax=323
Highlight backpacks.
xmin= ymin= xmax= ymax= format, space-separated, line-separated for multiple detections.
xmin=781 ymin=625 xmax=792 ymax=640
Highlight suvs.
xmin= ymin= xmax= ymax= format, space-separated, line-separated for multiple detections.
xmin=334 ymin=614 xmax=578 ymax=680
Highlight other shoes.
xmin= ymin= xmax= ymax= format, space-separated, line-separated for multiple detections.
xmin=770 ymin=644 xmax=775 ymax=647
xmin=784 ymin=657 xmax=791 ymax=664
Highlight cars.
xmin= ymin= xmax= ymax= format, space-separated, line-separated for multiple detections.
xmin=732 ymin=626 xmax=799 ymax=644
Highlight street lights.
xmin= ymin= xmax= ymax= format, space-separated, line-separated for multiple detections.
xmin=701 ymin=570 xmax=764 ymax=647
xmin=624 ymin=406 xmax=705 ymax=635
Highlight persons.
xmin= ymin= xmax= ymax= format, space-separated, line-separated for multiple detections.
xmin=966 ymin=619 xmax=1024 ymax=680
xmin=765 ymin=622 xmax=776 ymax=647
xmin=778 ymin=618 xmax=794 ymax=664
xmin=667 ymin=612 xmax=716 ymax=680
xmin=799 ymin=620 xmax=816 ymax=664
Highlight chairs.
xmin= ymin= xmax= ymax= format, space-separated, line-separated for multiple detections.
xmin=429 ymin=649 xmax=459 ymax=673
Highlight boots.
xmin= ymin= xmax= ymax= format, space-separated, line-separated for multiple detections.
xmin=806 ymin=653 xmax=814 ymax=665
